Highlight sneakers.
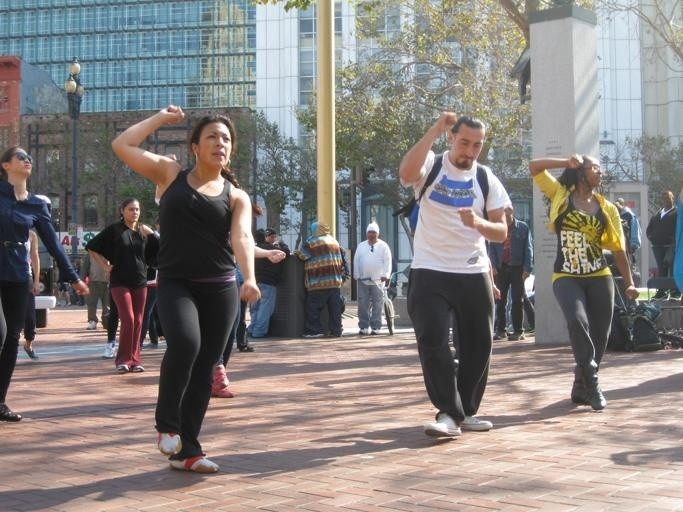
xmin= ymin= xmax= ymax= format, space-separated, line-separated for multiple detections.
xmin=651 ymin=288 xmax=668 ymax=301
xmin=104 ymin=343 xmax=115 ymax=358
xmin=461 ymin=415 xmax=492 ymax=430
xmin=158 ymin=432 xmax=182 ymax=454
xmin=210 ymin=387 xmax=232 ymax=398
xmin=86 ymin=321 xmax=96 ymax=329
xmin=493 ymin=329 xmax=505 ymax=340
xmin=301 ymin=329 xmax=325 ymax=337
xmin=508 ymin=331 xmax=525 ymax=341
xmin=572 ymin=374 xmax=605 ymax=410
xmin=170 ymin=456 xmax=219 ymax=473
xmin=668 ymin=290 xmax=682 ymax=302
xmin=117 ymin=365 xmax=130 ymax=371
xmin=424 ymin=413 xmax=462 ymax=436
xmin=213 ymin=364 xmax=229 ymax=390
xmin=131 ymin=365 xmax=144 ymax=372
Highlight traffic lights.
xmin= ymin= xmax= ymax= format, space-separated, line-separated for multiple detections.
xmin=362 ymin=163 xmax=375 ymax=184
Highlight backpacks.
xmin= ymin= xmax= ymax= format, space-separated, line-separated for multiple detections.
xmin=619 ymin=306 xmax=662 ymax=351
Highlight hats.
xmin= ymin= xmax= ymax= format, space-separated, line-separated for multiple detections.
xmin=367 ymin=223 xmax=379 ymax=233
xmin=372 ymin=329 xmax=378 ymax=334
xmin=359 ymin=328 xmax=365 ymax=334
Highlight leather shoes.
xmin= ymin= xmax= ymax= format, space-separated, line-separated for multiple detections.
xmin=0 ymin=404 xmax=20 ymax=421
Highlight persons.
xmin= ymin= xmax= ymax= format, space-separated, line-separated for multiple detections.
xmin=528 ymin=153 xmax=639 ymax=410
xmin=111 ymin=104 xmax=262 ymax=474
xmin=646 ymin=189 xmax=679 ymax=300
xmin=353 ymin=221 xmax=393 ymax=335
xmin=613 ymin=198 xmax=640 ymax=270
xmin=399 ymin=110 xmax=515 ymax=436
xmin=1 ymin=146 xmax=90 ymax=419
xmin=487 ymin=206 xmax=533 ymax=341
xmin=23 ymin=229 xmax=41 ymax=360
xmin=56 ymin=197 xmax=157 ymax=374
xmin=210 ymin=225 xmax=349 ymax=397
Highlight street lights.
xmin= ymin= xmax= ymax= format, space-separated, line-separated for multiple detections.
xmin=64 ymin=55 xmax=84 ymax=265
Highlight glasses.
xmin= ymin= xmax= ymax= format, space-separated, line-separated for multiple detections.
xmin=15 ymin=152 xmax=32 ymax=161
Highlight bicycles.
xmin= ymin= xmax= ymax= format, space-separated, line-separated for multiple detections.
xmin=381 ymin=273 xmax=407 ymax=335
